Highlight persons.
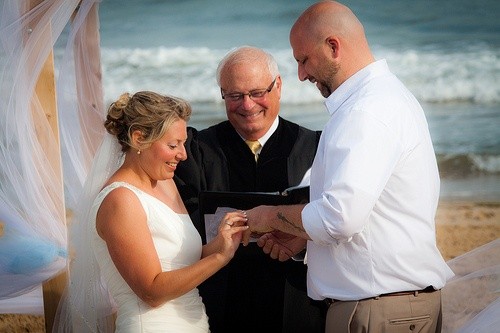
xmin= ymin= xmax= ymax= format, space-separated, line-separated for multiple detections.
xmin=174 ymin=46 xmax=323 ymax=333
xmin=241 ymin=0 xmax=455 ymax=333
xmin=51 ymin=90 xmax=249 ymax=333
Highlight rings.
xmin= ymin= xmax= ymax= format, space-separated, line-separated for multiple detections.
xmin=225 ymin=222 xmax=232 ymax=226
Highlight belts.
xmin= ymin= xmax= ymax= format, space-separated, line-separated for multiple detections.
xmin=324 ymin=285 xmax=435 ymax=306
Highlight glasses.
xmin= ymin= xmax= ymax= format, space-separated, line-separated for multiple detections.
xmin=221 ymin=78 xmax=276 ymax=101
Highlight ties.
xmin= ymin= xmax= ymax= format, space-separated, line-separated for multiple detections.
xmin=245 ymin=140 xmax=261 ymax=163
